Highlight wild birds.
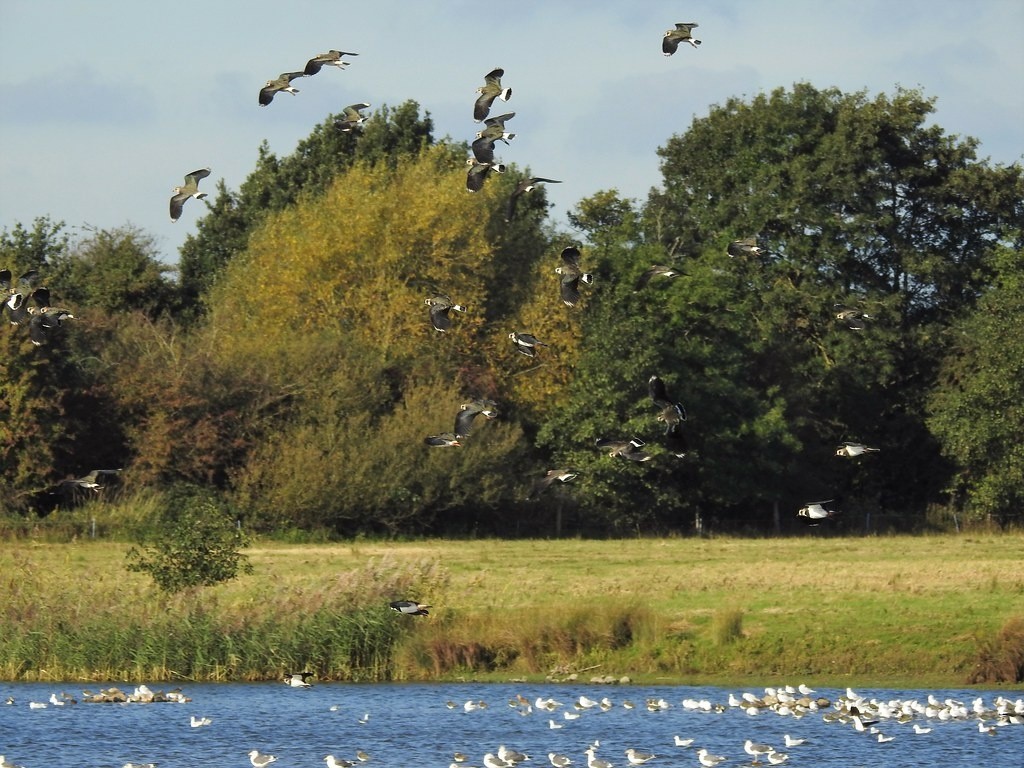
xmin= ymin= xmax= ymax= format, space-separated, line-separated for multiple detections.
xmin=0 ymin=671 xmax=1024 ymax=768
xmin=1 ymin=24 xmax=886 ymax=617
